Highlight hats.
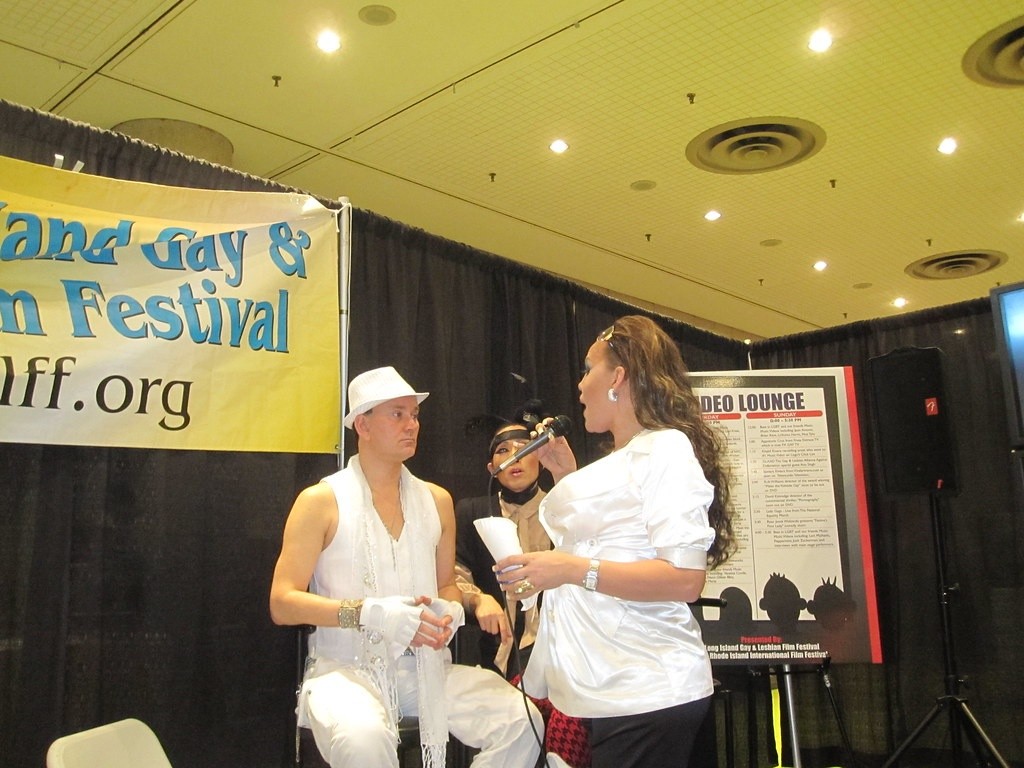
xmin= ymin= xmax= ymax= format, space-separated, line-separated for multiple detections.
xmin=343 ymin=366 xmax=430 ymax=430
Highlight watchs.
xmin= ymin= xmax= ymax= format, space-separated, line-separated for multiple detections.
xmin=583 ymin=557 xmax=601 ymax=591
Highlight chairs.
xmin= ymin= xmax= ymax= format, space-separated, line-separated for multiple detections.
xmin=46 ymin=717 xmax=173 ymax=768
xmin=295 ymin=627 xmax=420 ymax=764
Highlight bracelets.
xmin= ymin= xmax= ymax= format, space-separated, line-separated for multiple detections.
xmin=336 ymin=595 xmax=363 ymax=629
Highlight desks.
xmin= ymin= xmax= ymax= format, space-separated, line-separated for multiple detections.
xmin=712 ymin=655 xmax=826 ymax=768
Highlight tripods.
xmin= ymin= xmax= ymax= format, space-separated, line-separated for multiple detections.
xmin=883 ymin=496 xmax=1011 ymax=768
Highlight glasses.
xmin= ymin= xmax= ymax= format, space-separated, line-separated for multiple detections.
xmin=597 ymin=324 xmax=623 ymax=364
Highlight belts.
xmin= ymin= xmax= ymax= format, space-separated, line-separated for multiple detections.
xmin=304 ymin=646 xmax=417 ymax=669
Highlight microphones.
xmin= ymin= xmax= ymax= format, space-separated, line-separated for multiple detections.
xmin=493 ymin=415 xmax=573 ymax=476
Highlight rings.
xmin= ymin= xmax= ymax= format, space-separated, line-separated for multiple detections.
xmin=513 ymin=579 xmax=534 ymax=596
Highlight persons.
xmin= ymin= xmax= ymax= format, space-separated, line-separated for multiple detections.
xmin=491 ymin=315 xmax=741 ymax=768
xmin=453 ymin=398 xmax=595 ymax=768
xmin=269 ymin=365 xmax=544 ymax=768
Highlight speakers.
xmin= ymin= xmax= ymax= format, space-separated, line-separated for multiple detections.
xmin=866 ymin=347 xmax=957 ymax=499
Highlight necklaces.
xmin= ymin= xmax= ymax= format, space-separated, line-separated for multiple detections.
xmin=612 ymin=428 xmax=646 ymax=452
